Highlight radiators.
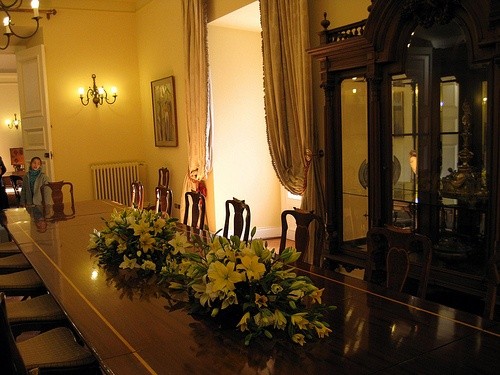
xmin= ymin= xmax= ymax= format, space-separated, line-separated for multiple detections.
xmin=90 ymin=162 xmax=144 ymax=207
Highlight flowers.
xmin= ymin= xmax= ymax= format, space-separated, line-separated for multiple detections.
xmin=87 ymin=207 xmax=338 ymax=347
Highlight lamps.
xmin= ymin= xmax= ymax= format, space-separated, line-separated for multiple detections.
xmin=0 ymin=0 xmax=57 ymax=50
xmin=78 ymin=74 xmax=118 ymax=108
xmin=6 ymin=114 xmax=19 ymax=129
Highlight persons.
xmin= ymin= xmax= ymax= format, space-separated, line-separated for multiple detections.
xmin=20 ymin=157 xmax=55 ymax=205
xmin=26 ymin=204 xmax=54 ymax=233
xmin=0 ymin=157 xmax=9 ymax=219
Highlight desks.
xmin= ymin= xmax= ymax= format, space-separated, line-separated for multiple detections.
xmin=3 ymin=198 xmax=500 ymax=375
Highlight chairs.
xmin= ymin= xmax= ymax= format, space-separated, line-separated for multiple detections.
xmin=40 ymin=180 xmax=74 ymax=204
xmin=9 ymin=175 xmax=23 ymax=208
xmin=130 ymin=167 xmax=433 ymax=299
xmin=0 ymin=240 xmax=97 ymax=375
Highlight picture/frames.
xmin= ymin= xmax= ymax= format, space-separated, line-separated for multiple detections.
xmin=151 ymin=76 xmax=178 ymax=147
